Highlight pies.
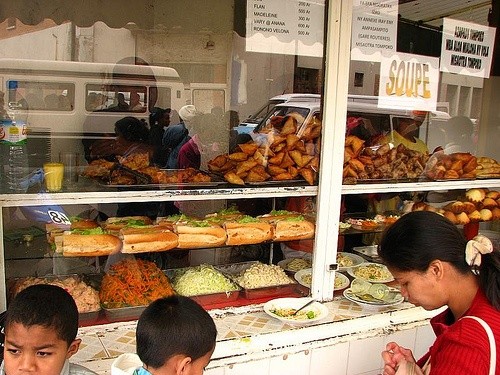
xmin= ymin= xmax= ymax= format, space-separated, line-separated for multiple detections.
xmin=79 ymin=151 xmax=213 ymax=184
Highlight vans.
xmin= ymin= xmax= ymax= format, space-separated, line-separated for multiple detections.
xmin=231 ymin=92 xmax=454 ymax=176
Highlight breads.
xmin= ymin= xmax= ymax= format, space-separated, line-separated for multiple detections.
xmin=342 ymin=135 xmax=500 ymax=225
xmin=223 ymin=113 xmax=321 ymax=186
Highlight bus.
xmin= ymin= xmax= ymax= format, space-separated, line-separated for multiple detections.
xmin=0 ymin=58 xmax=188 ymax=231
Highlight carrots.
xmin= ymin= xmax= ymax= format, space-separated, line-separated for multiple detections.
xmin=98 ymin=258 xmax=174 ymax=309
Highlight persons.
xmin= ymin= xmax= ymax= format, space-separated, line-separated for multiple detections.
xmin=377 ymin=210 xmax=500 ymax=375
xmin=82 ymin=57 xmax=191 ymax=270
xmin=133 ymin=294 xmax=217 ymax=375
xmin=21 ymin=90 xmax=248 ymax=213
xmin=280 ymin=195 xmax=348 ymax=256
xmin=0 ymin=284 xmax=99 ymax=375
xmin=351 ymin=110 xmax=479 ymax=205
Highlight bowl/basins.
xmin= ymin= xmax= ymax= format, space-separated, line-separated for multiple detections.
xmin=263 ymin=297 xmax=328 ymax=326
xmin=277 ymin=258 xmax=312 ymax=273
xmin=343 ymin=286 xmax=405 ymax=310
xmin=294 ymin=268 xmax=350 ymax=291
xmin=337 ymin=251 xmax=364 ymax=272
xmin=347 ymin=263 xmax=395 ymax=283
xmin=340 ymin=222 xmax=351 ymax=232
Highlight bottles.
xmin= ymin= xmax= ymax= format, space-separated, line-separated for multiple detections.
xmin=0 ymin=80 xmax=29 ymax=193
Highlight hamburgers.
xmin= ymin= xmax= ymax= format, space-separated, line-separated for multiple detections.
xmin=46 ymin=206 xmax=315 ymax=257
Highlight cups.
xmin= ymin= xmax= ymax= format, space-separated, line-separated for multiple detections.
xmin=43 ymin=162 xmax=63 ymax=191
